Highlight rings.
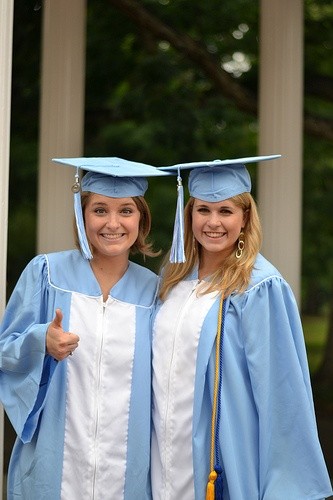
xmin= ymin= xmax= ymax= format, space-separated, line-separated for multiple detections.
xmin=69 ymin=351 xmax=72 ymax=356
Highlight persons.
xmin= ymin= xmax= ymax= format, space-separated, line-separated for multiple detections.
xmin=149 ymin=155 xmax=333 ymax=500
xmin=0 ymin=157 xmax=179 ymax=500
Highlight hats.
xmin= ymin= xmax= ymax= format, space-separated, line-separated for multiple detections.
xmin=155 ymin=155 xmax=282 ymax=264
xmin=51 ymin=157 xmax=172 ymax=261
